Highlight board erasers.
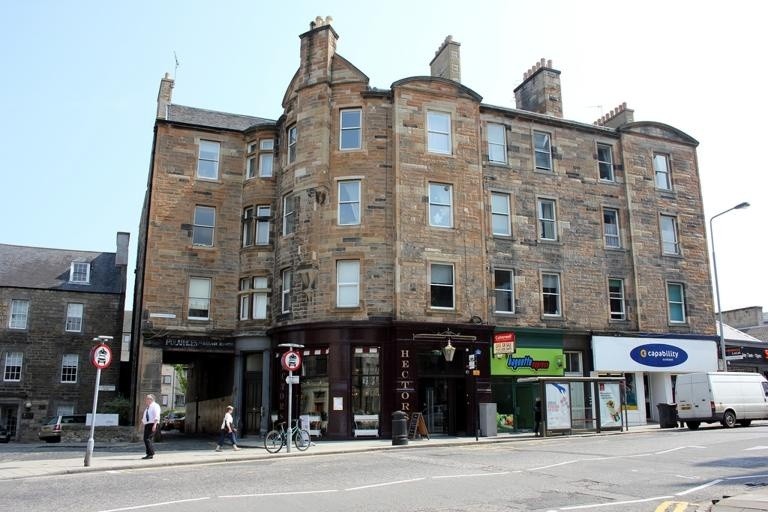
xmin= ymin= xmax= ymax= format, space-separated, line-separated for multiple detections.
xmin=656 ymin=403 xmax=677 ymax=428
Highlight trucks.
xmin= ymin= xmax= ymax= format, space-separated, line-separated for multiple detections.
xmin=675 ymin=371 xmax=768 ymax=430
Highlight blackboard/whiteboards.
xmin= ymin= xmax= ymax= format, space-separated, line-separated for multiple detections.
xmin=407 ymin=412 xmax=420 ymax=440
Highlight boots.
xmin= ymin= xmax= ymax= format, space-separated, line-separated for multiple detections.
xmin=233 ymin=444 xmax=240 ymax=451
xmin=215 ymin=445 xmax=223 ymax=452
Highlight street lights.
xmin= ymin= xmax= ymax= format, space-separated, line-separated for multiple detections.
xmin=709 ymin=203 xmax=748 ymax=371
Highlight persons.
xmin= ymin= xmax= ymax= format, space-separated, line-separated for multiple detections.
xmin=215 ymin=405 xmax=242 ymax=452
xmin=138 ymin=394 xmax=161 ymax=459
xmin=533 ymin=396 xmax=544 ymax=438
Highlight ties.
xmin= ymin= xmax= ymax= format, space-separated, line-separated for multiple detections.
xmin=146 ymin=406 xmax=149 ymax=422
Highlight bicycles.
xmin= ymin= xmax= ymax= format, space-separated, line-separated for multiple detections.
xmin=264 ymin=419 xmax=310 ymax=453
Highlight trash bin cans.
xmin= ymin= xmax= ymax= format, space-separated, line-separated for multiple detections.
xmin=391 ymin=411 xmax=408 ymax=445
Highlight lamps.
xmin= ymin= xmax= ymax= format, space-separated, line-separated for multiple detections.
xmin=444 ymin=340 xmax=457 ymax=362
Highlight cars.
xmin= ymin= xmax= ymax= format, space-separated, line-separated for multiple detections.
xmin=0 ymin=424 xmax=10 ymax=443
xmin=38 ymin=414 xmax=85 ymax=443
xmin=160 ymin=413 xmax=185 ymax=432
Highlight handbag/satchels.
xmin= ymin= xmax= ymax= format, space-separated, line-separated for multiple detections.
xmin=225 ymin=422 xmax=234 ymax=432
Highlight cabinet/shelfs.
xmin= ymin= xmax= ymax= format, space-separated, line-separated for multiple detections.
xmin=299 ymin=415 xmax=321 ymax=440
xmin=354 ymin=414 xmax=379 ymax=438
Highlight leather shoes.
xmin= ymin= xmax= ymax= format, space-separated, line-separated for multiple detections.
xmin=142 ymin=454 xmax=153 ymax=459
